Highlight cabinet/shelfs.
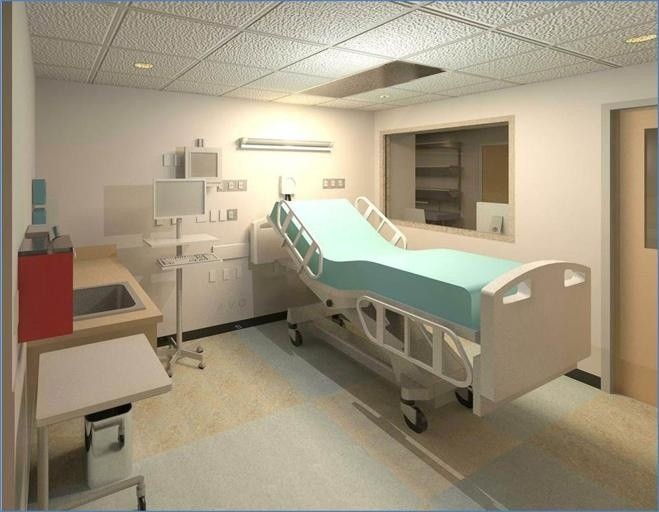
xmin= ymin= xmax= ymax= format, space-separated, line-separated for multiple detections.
xmin=416 ymin=143 xmax=462 ymax=226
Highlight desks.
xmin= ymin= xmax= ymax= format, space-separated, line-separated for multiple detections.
xmin=35 ymin=333 xmax=172 ymax=512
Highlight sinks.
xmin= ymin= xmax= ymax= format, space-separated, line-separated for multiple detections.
xmin=73 ymin=281 xmax=147 ymax=321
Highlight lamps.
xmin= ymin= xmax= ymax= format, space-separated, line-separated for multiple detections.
xmin=240 ymin=137 xmax=334 ymax=153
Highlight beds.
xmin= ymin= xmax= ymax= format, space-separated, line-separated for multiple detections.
xmin=266 ymin=196 xmax=591 ymax=434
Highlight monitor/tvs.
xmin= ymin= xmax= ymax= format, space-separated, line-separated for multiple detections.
xmin=153 ymin=178 xmax=206 ymax=219
xmin=184 ymin=147 xmax=220 ymax=179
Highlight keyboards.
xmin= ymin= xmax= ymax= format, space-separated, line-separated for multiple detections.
xmin=158 ymin=253 xmax=222 ymax=267
